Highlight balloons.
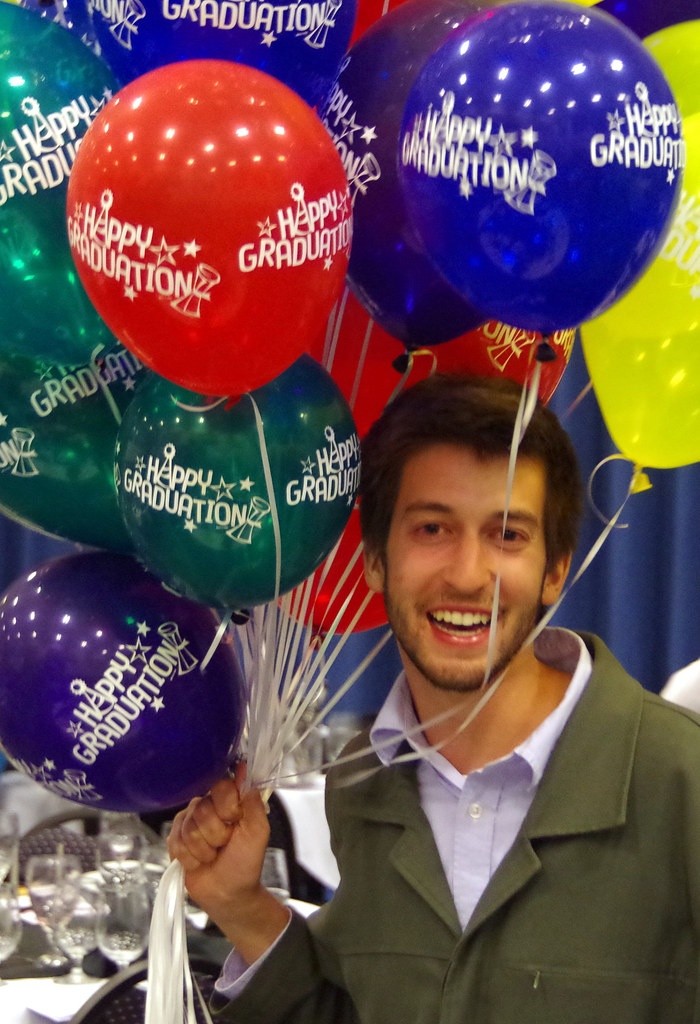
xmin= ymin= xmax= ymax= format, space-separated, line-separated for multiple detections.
xmin=0 ymin=0 xmax=700 ymax=818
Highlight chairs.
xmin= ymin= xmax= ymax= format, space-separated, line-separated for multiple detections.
xmin=21 ymin=790 xmax=299 ymax=1021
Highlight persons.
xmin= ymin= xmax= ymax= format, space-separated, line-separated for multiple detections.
xmin=163 ymin=372 xmax=700 ymax=1023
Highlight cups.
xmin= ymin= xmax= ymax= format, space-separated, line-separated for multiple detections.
xmin=0 ymin=812 xmax=20 ymax=884
xmin=260 ymin=848 xmax=289 ymax=902
xmin=0 ymin=886 xmax=23 ymax=962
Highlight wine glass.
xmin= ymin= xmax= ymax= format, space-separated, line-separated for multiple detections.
xmin=27 ymin=855 xmax=80 ymax=952
xmin=97 ymin=887 xmax=152 ymax=974
xmin=97 ymin=832 xmax=144 ymax=896
xmin=59 ymin=905 xmax=96 ymax=984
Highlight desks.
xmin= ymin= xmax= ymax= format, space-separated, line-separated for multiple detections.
xmin=0 ymin=860 xmax=322 ymax=1024
